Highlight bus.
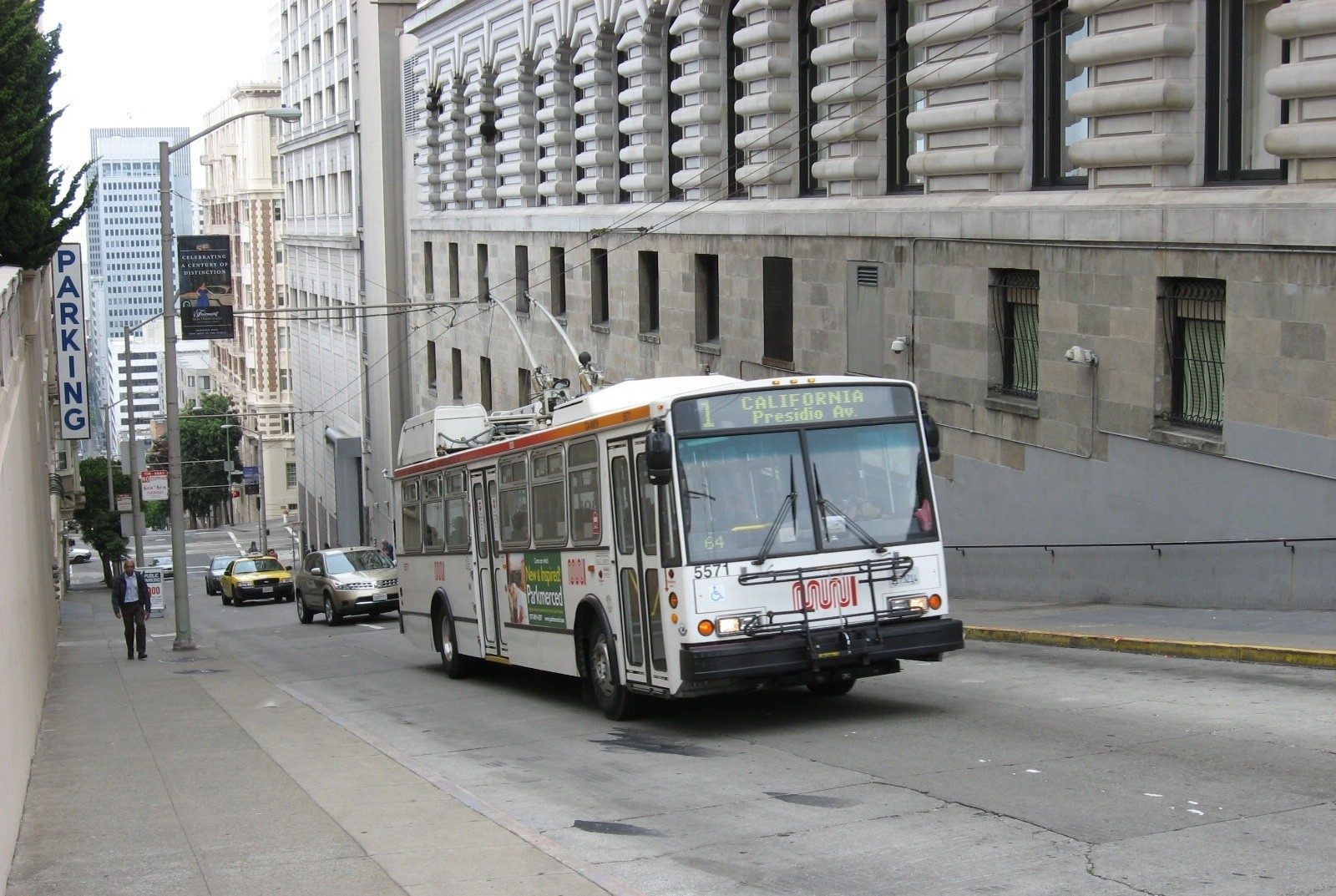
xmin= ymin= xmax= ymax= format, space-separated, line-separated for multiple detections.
xmin=378 ymin=295 xmax=971 ymax=721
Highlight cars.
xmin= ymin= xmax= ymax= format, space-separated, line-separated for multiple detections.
xmin=219 ymin=551 xmax=296 ymax=607
xmin=148 ymin=558 xmax=176 ymax=580
xmin=68 ymin=546 xmax=92 ymax=564
xmin=294 ymin=545 xmax=403 ymax=627
xmin=203 ymin=554 xmax=242 ymax=596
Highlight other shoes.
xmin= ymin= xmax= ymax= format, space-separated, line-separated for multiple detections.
xmin=138 ymin=652 xmax=147 ymax=658
xmin=128 ymin=648 xmax=134 ymax=659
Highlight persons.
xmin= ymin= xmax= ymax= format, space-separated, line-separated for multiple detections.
xmin=111 ymin=559 xmax=152 ymax=659
xmin=250 ymin=541 xmax=278 ymax=559
xmin=425 ymin=512 xmax=529 ymax=547
xmin=381 ymin=540 xmax=394 ymax=560
xmin=822 ymin=466 xmax=881 ymax=521
xmin=195 ymin=281 xmax=216 ymax=307
xmin=307 ymin=543 xmax=331 ymax=552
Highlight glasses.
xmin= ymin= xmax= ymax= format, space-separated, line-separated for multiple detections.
xmin=126 ymin=566 xmax=134 ymax=568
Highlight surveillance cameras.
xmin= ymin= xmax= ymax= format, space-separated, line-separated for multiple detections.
xmin=891 ymin=341 xmax=904 ymax=354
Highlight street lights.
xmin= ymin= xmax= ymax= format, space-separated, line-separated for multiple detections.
xmin=220 ymin=424 xmax=268 ymax=557
xmin=160 ymin=105 xmax=305 ymax=655
xmin=193 ymin=406 xmax=235 ymax=528
